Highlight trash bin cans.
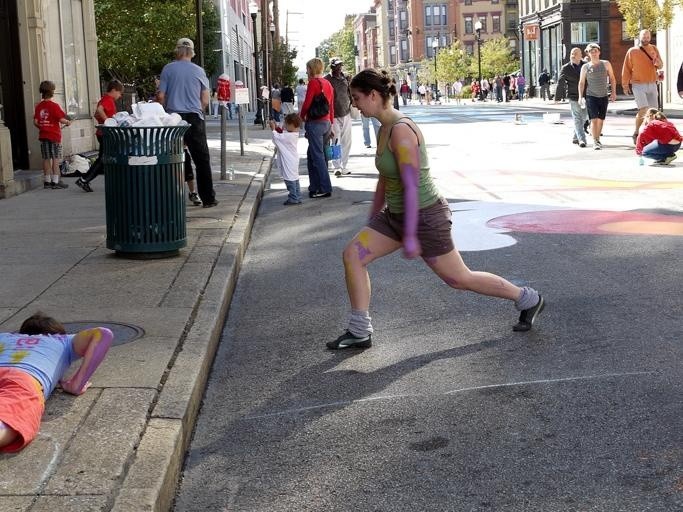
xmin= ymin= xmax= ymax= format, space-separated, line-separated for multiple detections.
xmin=94 ymin=124 xmax=193 ymax=260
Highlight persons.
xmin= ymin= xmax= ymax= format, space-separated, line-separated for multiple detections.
xmin=578 ymin=43 xmax=617 ymax=149
xmin=216 ymin=73 xmax=233 ymax=120
xmin=264 ymin=55 xmax=383 ymax=206
xmin=182 ymin=144 xmax=203 ymax=206
xmin=388 ymin=68 xmax=554 ymax=110
xmin=210 ymin=88 xmax=219 ymax=119
xmin=156 ymin=36 xmax=221 ymax=208
xmin=75 ymin=78 xmax=125 ymax=192
xmin=635 ymin=107 xmax=683 ymax=166
xmin=325 ymin=69 xmax=547 ymax=350
xmin=584 ymin=55 xmax=604 ymax=136
xmin=620 ymin=28 xmax=665 ymax=145
xmin=32 ymin=79 xmax=73 ymax=190
xmin=676 ymin=61 xmax=683 ymax=100
xmin=555 ymin=46 xmax=589 ymax=148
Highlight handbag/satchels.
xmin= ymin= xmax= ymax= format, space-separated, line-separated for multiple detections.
xmin=307 ymin=78 xmax=328 ymax=119
xmin=324 ymin=137 xmax=341 ymax=161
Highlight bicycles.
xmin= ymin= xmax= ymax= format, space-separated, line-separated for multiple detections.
xmin=253 ymin=95 xmax=271 ymax=132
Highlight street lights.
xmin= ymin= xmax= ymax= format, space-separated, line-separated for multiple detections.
xmin=430 ymin=34 xmax=442 ymax=104
xmin=472 ymin=19 xmax=484 ymax=102
xmin=248 ymin=0 xmax=264 ymax=124
xmin=285 ymin=8 xmax=306 ymax=53
xmin=270 ymin=20 xmax=276 ymax=70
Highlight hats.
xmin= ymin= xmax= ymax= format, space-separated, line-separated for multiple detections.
xmin=175 ymin=38 xmax=194 ymax=50
xmin=329 ymin=58 xmax=344 ymax=68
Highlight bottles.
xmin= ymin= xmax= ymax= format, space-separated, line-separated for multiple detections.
xmin=580 ymin=97 xmax=586 ymax=109
xmin=639 ymin=157 xmax=644 ymax=166
xmin=228 ymin=163 xmax=235 ymax=181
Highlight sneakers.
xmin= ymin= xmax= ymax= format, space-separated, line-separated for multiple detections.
xmin=632 ymin=134 xmax=638 ymax=144
xmin=51 ymin=181 xmax=67 ymax=189
xmin=283 ymin=167 xmax=351 ymax=206
xmin=43 ymin=182 xmax=52 ymax=189
xmin=74 ymin=179 xmax=92 ymax=192
xmin=188 ymin=192 xmax=202 ymax=206
xmin=572 ymin=130 xmax=602 ymax=150
xmin=512 ymin=294 xmax=544 ymax=331
xmin=659 ymin=154 xmax=677 ymax=165
xmin=325 ymin=328 xmax=372 ymax=350
xmin=366 ymin=144 xmax=371 ymax=148
xmin=203 ymin=199 xmax=217 ymax=208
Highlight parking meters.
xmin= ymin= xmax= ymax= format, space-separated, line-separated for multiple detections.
xmin=215 ymin=72 xmax=231 ymax=181
xmin=234 ymin=80 xmax=246 ymax=158
xmin=658 ymin=70 xmax=664 ymax=114
